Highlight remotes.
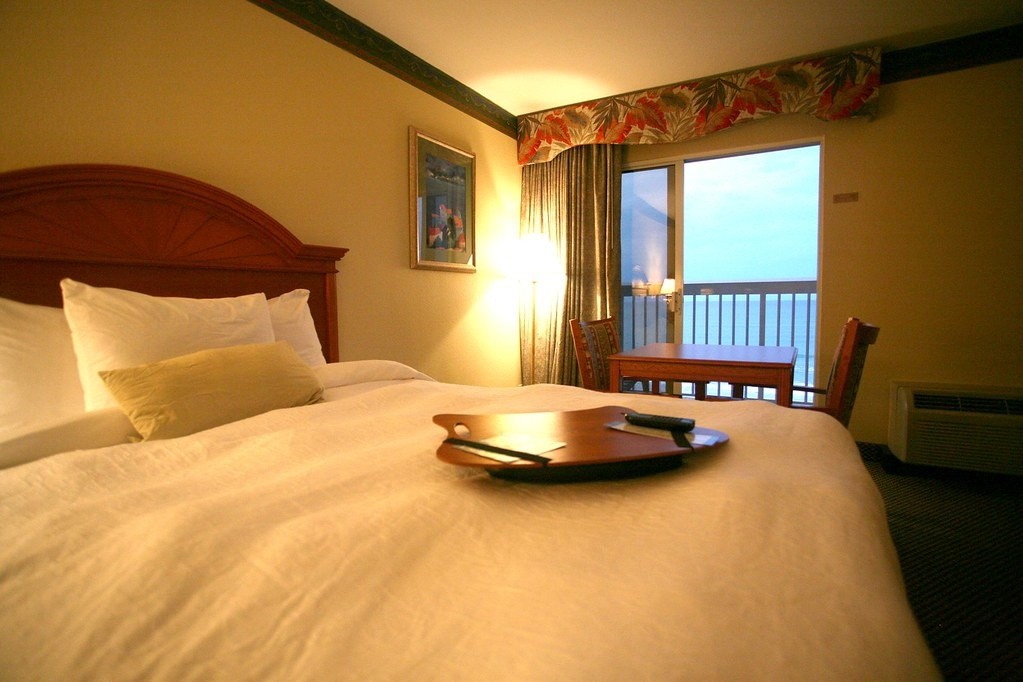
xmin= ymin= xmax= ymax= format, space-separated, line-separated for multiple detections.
xmin=623 ymin=412 xmax=694 ymax=432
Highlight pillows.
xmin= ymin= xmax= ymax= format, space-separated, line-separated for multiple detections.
xmin=0 ymin=276 xmax=327 ymax=444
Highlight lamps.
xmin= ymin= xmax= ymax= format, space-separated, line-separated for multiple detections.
xmin=660 ymin=278 xmax=678 ymax=303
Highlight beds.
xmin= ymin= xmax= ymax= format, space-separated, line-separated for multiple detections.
xmin=0 ymin=164 xmax=946 ymax=682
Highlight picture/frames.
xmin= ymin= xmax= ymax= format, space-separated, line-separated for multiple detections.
xmin=408 ymin=124 xmax=478 ymax=272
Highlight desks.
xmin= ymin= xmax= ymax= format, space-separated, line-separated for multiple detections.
xmin=606 ymin=343 xmax=798 ymax=408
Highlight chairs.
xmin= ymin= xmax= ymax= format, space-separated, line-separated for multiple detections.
xmin=569 ymin=313 xmax=880 ymax=428
